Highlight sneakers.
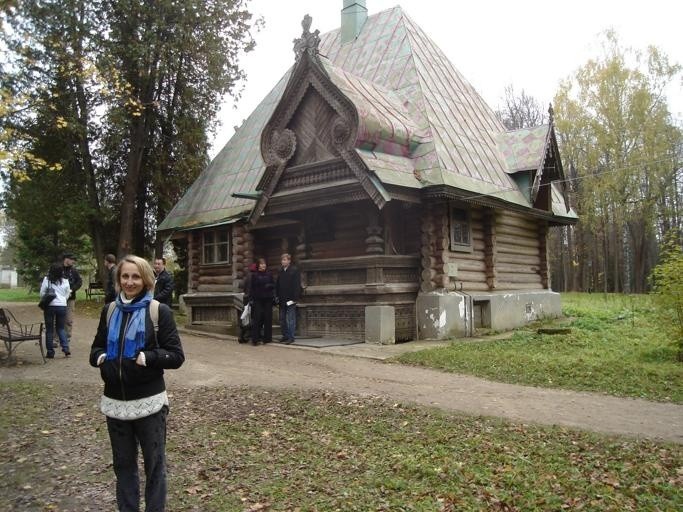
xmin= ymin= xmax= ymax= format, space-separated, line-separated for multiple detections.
xmin=56 ymin=333 xmax=71 ymax=342
xmin=61 ymin=346 xmax=71 ymax=356
xmin=46 ymin=349 xmax=55 ymax=358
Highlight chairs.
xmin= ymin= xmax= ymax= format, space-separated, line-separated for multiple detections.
xmin=0 ymin=307 xmax=47 ymax=367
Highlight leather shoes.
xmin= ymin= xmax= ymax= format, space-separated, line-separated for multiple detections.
xmin=279 ymin=334 xmax=295 ymax=344
xmin=238 ymin=334 xmax=273 ymax=346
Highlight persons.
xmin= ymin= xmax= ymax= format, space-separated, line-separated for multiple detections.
xmin=153 ymin=256 xmax=177 ymax=308
xmin=89 ymin=254 xmax=185 ymax=512
xmin=238 ymin=257 xmax=275 ymax=344
xmin=53 ymin=253 xmax=82 ymax=342
xmin=275 ymin=253 xmax=302 ymax=345
xmin=39 ymin=262 xmax=72 ymax=358
xmin=103 ymin=254 xmax=117 ymax=304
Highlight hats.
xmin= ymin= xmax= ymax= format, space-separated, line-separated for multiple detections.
xmin=63 ymin=253 xmax=76 ymax=259
xmin=248 ymin=263 xmax=256 ymax=271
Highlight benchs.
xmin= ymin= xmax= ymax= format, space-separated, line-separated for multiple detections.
xmin=85 ymin=282 xmax=105 ymax=301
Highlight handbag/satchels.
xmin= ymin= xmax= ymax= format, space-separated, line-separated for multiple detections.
xmin=38 ymin=288 xmax=56 ymax=310
xmin=240 ymin=302 xmax=252 ymax=327
